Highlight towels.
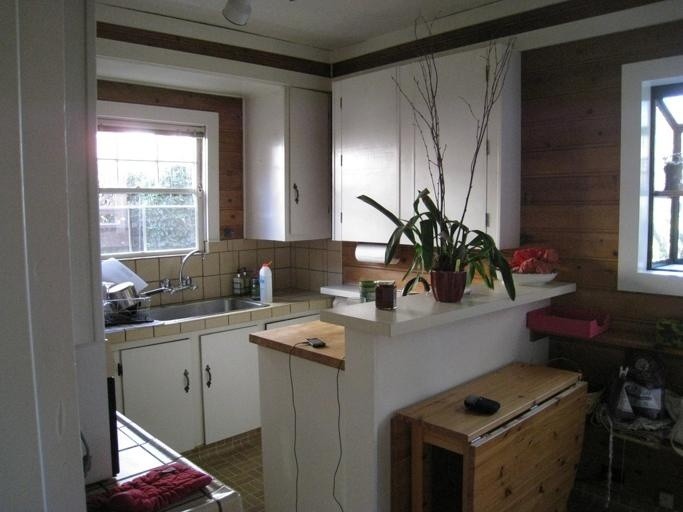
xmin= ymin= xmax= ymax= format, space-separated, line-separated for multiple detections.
xmin=86 ymin=462 xmax=213 ymax=511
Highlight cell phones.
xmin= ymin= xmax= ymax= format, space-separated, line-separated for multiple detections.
xmin=306 ymin=337 xmax=326 ymax=348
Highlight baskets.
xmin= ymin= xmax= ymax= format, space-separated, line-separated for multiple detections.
xmin=104 ymin=297 xmax=152 ymax=325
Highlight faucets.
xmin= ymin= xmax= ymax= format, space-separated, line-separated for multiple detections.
xmin=179 ymin=250 xmax=205 ymax=286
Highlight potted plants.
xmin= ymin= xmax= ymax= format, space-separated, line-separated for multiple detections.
xmin=350 ymin=191 xmax=524 ymax=303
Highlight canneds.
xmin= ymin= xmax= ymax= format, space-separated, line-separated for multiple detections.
xmin=375 ymin=280 xmax=396 ymax=311
xmin=359 ymin=280 xmax=374 ymax=304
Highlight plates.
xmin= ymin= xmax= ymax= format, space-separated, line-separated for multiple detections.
xmin=496 ymin=271 xmax=558 ymax=286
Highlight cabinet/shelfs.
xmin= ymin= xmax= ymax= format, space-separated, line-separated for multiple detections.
xmin=397 ymin=361 xmax=589 ymax=512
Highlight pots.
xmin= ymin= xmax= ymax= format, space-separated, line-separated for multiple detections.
xmin=107 ymin=281 xmax=169 ymax=310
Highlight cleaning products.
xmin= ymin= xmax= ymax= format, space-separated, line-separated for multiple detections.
xmin=259 ymin=260 xmax=273 ymax=305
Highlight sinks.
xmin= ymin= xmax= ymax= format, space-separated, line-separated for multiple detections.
xmin=151 ymin=297 xmax=270 ymax=324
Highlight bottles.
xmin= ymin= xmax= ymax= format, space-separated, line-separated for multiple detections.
xmin=233 ymin=263 xmax=272 ymax=303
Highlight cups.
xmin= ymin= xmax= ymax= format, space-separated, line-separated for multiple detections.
xmin=376 ymin=284 xmax=394 ymax=311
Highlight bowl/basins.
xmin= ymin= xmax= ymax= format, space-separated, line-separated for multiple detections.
xmin=587 ymin=381 xmax=605 ymax=416
